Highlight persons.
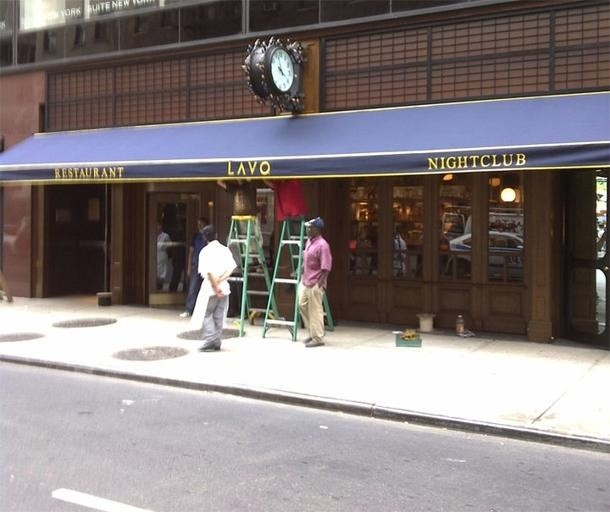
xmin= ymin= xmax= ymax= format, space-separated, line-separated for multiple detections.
xmin=156 ymin=231 xmax=178 ymax=291
xmin=190 ymin=224 xmax=237 ymax=350
xmin=299 ymin=217 xmax=332 ymax=347
xmin=179 ymin=217 xmax=208 ymax=318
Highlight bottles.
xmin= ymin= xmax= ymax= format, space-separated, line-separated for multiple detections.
xmin=456 ymin=314 xmax=464 ymax=336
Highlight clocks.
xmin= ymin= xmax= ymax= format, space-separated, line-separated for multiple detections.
xmin=246 ymin=36 xmax=305 ymax=116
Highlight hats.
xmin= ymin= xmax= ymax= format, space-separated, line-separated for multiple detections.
xmin=304 ymin=217 xmax=325 ymax=229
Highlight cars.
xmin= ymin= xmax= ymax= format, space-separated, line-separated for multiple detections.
xmin=352 ymin=199 xmax=524 ymax=279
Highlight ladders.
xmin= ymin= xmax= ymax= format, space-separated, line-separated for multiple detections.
xmin=263 ymin=218 xmax=334 ymax=342
xmin=227 ymin=216 xmax=281 ymax=337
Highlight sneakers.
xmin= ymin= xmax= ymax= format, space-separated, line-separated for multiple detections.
xmin=198 ymin=342 xmax=221 ymax=352
xmin=179 ymin=312 xmax=191 ymax=318
xmin=303 ymin=338 xmax=325 ymax=347
